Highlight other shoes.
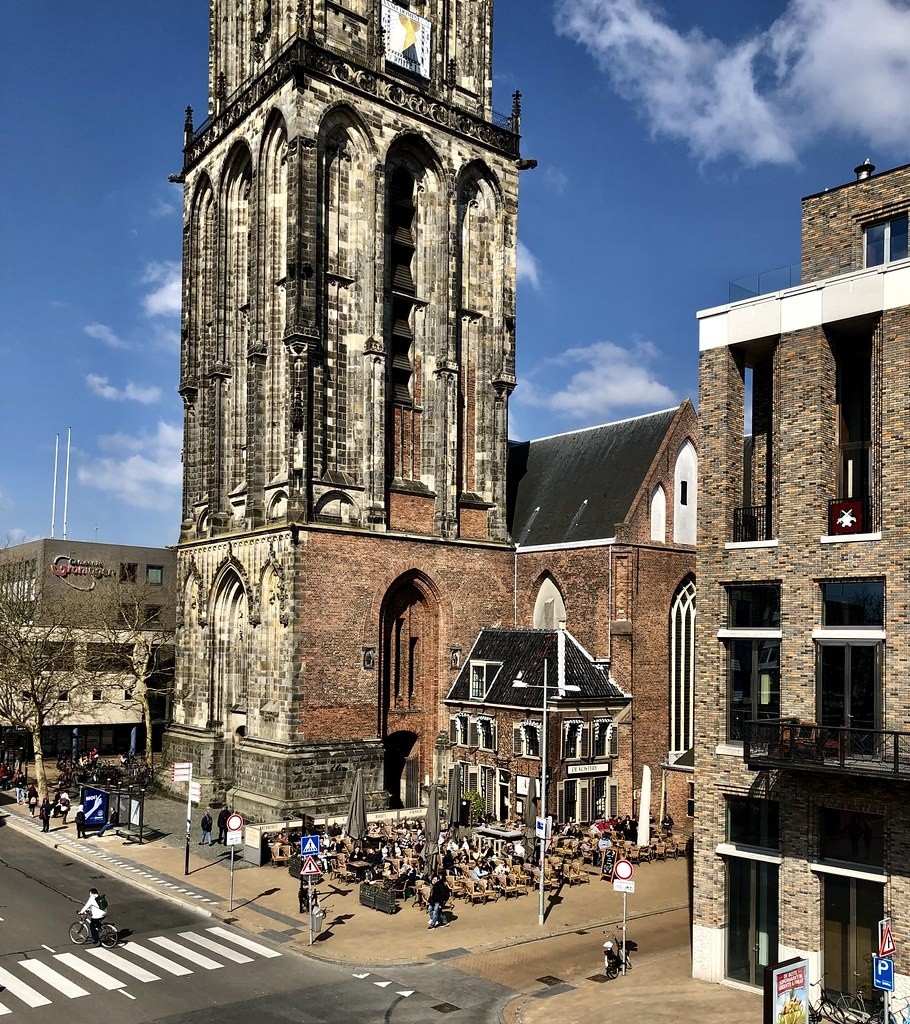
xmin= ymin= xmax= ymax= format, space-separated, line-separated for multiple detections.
xmin=44 ymin=830 xmax=48 ymax=832
xmin=78 ymin=837 xmax=82 ymax=838
xmin=41 ymin=830 xmax=45 ymax=832
xmin=98 ymin=834 xmax=101 ymax=836
xmin=84 ymin=836 xmax=89 ymax=838
xmin=209 ymin=844 xmax=212 ymax=846
xmin=93 ymin=939 xmax=99 ymax=944
xmin=199 ymin=842 xmax=204 ymax=845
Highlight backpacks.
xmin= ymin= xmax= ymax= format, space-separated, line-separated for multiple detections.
xmin=95 ymin=893 xmax=108 ymax=910
xmin=440 ymin=885 xmax=450 ymax=902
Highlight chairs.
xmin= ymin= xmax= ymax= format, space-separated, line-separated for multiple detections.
xmin=768 ymin=721 xmax=818 ymax=756
xmin=263 ymin=814 xmax=688 ymax=914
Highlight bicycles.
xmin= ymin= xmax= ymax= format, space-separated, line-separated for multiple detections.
xmin=808 ymin=973 xmax=846 ymax=1024
xmin=603 ymin=924 xmax=633 ymax=979
xmin=833 ymin=986 xmax=889 ymax=1024
xmin=866 ymin=994 xmax=910 ymax=1023
xmin=69 ymin=909 xmax=119 ymax=948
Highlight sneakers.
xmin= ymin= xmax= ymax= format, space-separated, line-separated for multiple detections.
xmin=438 ymin=923 xmax=449 ymax=927
xmin=427 ymin=926 xmax=434 ymax=930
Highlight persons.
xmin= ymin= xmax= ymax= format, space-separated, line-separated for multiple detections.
xmin=41 ymin=799 xmax=53 ymax=834
xmin=77 ymin=889 xmax=109 ymax=947
xmin=0 ymin=747 xmax=131 ymax=791
xmin=200 ymin=809 xmax=215 ymax=847
xmin=263 ymin=812 xmax=696 ymax=897
xmin=97 ymin=807 xmax=119 ymax=839
xmin=59 ymin=791 xmax=72 ymax=827
xmin=49 ymin=790 xmax=62 ymax=817
xmin=14 ymin=772 xmax=28 ymax=808
xmin=217 ymin=806 xmax=232 ymax=846
xmin=428 ymin=878 xmax=441 ymax=927
xmin=25 ymin=783 xmax=40 ymax=818
xmin=76 ymin=804 xmax=90 ymax=839
xmin=429 ymin=876 xmax=451 ymax=931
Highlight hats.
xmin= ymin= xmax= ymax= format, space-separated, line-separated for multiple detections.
xmin=78 ymin=805 xmax=84 ymax=812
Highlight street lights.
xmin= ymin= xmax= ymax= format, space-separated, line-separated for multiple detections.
xmin=511 ymin=658 xmax=581 ymax=925
